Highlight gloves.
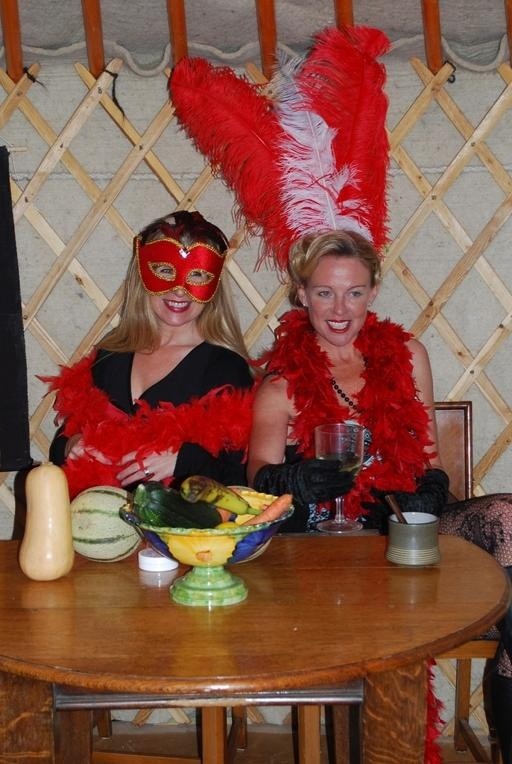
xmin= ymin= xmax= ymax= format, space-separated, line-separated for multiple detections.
xmin=358 ymin=468 xmax=449 ymax=535
xmin=253 ymin=457 xmax=354 ymax=504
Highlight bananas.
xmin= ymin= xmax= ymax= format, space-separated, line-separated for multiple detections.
xmin=179 ymin=473 xmax=263 ymax=517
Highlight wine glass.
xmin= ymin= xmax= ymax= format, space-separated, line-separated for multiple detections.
xmin=120 ymin=489 xmax=294 ymax=607
xmin=314 ymin=423 xmax=364 ymax=534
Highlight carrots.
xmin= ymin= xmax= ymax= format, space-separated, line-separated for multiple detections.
xmin=239 ymin=493 xmax=293 ymax=527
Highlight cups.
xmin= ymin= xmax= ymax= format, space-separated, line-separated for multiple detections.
xmin=385 ymin=512 xmax=441 ymax=569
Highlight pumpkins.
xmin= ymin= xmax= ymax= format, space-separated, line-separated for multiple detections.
xmin=18 ymin=461 xmax=75 ymax=582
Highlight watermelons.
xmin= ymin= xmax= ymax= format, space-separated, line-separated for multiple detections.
xmin=71 ymin=486 xmax=141 ymax=563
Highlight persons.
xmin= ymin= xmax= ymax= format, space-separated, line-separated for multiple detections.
xmin=246 ymin=228 xmax=511 ymax=764
xmin=48 ymin=210 xmax=254 ymax=500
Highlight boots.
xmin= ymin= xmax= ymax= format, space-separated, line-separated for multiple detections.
xmin=491 ymin=565 xmax=512 ymax=764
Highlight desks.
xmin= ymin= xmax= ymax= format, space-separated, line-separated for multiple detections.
xmin=1 ymin=528 xmax=512 ymax=764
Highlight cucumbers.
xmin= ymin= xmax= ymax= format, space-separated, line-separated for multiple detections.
xmin=132 ymin=479 xmax=222 ymax=528
